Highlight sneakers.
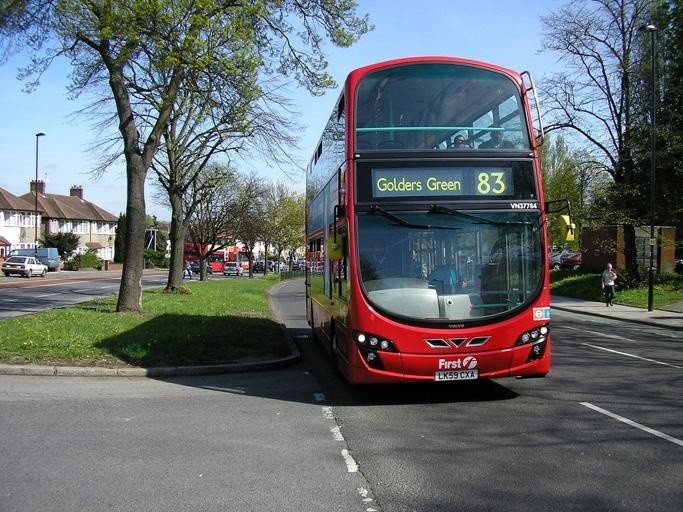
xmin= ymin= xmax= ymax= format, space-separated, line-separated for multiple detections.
xmin=606 ymin=301 xmax=613 ymax=307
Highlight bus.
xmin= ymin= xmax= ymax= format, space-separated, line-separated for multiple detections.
xmin=302 ymin=54 xmax=571 ymax=386
xmin=182 ymin=232 xmax=237 ymax=272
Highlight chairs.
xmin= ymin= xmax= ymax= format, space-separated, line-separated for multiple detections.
xmin=357 ymin=139 xmax=404 ymax=151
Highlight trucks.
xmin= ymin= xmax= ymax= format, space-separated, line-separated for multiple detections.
xmin=4 ymin=247 xmax=59 ymax=269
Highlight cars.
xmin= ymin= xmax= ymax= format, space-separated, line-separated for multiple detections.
xmin=251 ymin=259 xmax=288 ymax=274
xmin=223 ymin=261 xmax=244 ymax=276
xmin=0 ymin=255 xmax=49 ymax=278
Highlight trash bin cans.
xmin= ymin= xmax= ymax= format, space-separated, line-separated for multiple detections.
xmin=105 ymin=260 xmax=109 ymax=270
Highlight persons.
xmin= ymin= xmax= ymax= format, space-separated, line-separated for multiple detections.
xmin=452 ymin=135 xmax=466 ymax=148
xmin=363 ymin=238 xmax=485 ymax=314
xmin=267 ymin=258 xmax=292 ymax=274
xmin=601 ymin=262 xmax=617 ymax=307
xmin=476 ymin=122 xmax=515 ymax=150
xmin=183 ymin=259 xmax=192 ymax=279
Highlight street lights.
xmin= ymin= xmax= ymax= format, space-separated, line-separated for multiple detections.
xmin=34 ymin=131 xmax=45 ymax=255
